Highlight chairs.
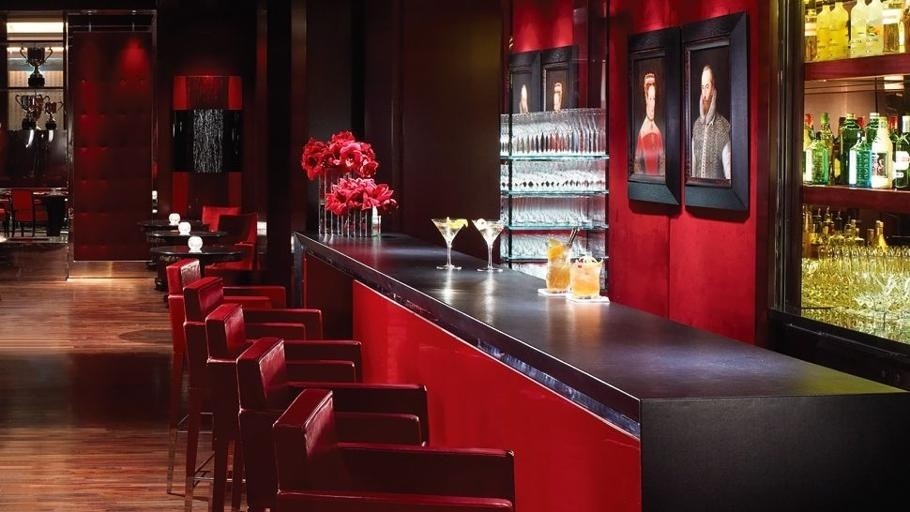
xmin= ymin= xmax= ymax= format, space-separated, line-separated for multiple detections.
xmin=274 ymin=387 xmax=516 ymax=512
xmin=166 ymin=255 xmax=287 ymax=491
xmin=203 ymin=212 xmax=264 ymax=284
xmin=0 ymin=188 xmax=69 ymax=237
xmin=236 ymin=336 xmax=429 ymax=512
xmin=183 ymin=277 xmax=323 ymax=511
xmin=205 ymin=304 xmax=362 ymax=511
xmin=200 ymin=204 xmax=241 ymax=232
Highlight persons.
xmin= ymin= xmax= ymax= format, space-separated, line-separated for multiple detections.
xmin=512 ymin=83 xmax=534 ymax=153
xmin=690 ymin=63 xmax=731 ymax=181
xmin=633 ymin=71 xmax=665 ymax=177
xmin=545 ymin=82 xmax=567 ymax=150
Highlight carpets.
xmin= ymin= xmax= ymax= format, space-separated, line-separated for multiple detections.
xmin=0 ymin=242 xmax=70 ymax=282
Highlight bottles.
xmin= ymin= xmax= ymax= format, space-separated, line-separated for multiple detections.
xmin=805 ymin=0 xmax=904 ymax=62
xmin=802 ymin=202 xmax=888 ymax=259
xmin=804 ymin=111 xmax=910 ymax=189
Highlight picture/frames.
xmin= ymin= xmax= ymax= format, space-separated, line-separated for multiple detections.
xmin=681 ymin=12 xmax=749 ymax=212
xmin=626 ymin=25 xmax=682 ymax=206
xmin=509 ymin=49 xmax=544 ymax=114
xmin=540 ymin=45 xmax=580 ymax=110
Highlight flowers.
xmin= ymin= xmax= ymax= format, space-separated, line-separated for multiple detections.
xmin=299 ymin=128 xmax=400 ymax=217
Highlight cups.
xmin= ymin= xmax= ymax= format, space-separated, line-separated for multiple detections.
xmin=545 ymin=242 xmax=603 ymax=298
xmin=500 ymin=193 xmax=606 ymax=258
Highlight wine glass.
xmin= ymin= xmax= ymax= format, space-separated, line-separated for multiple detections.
xmin=500 ymin=108 xmax=606 ymax=191
xmin=431 ymin=217 xmax=506 ymax=273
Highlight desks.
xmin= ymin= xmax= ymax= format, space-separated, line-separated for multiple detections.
xmin=134 ymin=215 xmax=246 ymax=309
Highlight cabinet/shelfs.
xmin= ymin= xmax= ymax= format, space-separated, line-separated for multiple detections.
xmin=5 ymin=38 xmax=66 ymax=183
xmin=776 ymin=0 xmax=910 ymax=385
